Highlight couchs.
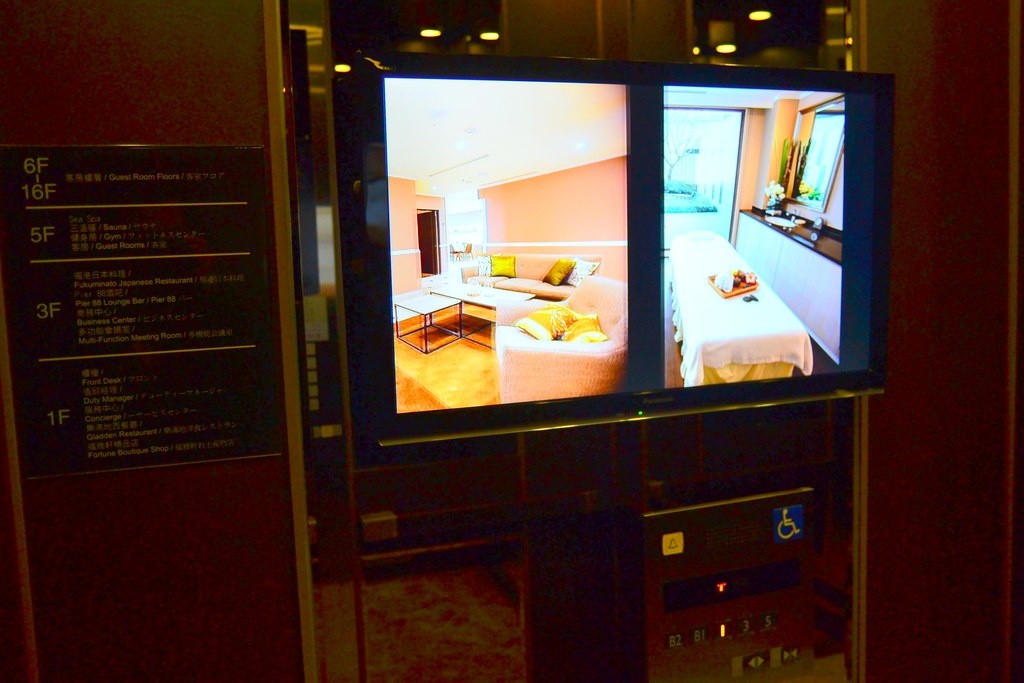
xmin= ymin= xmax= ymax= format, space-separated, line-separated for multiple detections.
xmin=497 ymin=278 xmax=628 ymax=403
xmin=461 ymin=256 xmax=601 ymax=301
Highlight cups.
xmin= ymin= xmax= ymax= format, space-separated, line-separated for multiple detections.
xmin=715 ymin=272 xmax=735 ymax=291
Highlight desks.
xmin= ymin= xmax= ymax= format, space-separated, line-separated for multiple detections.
xmin=394 ymin=299 xmax=463 ymax=355
xmin=669 ymin=231 xmax=813 ymax=388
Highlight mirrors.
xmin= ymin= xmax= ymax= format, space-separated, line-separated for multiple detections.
xmin=790 ymin=109 xmax=846 ymax=213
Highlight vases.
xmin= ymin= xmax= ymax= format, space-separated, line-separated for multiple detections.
xmin=768 ymin=200 xmax=780 ymax=210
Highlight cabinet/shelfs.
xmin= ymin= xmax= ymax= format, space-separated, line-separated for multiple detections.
xmin=735 ymin=214 xmax=843 ymax=365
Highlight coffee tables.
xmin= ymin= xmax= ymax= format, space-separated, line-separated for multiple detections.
xmin=430 ymin=285 xmax=538 ymax=349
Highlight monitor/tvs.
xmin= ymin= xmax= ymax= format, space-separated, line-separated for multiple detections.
xmin=349 ymin=49 xmax=894 ymax=440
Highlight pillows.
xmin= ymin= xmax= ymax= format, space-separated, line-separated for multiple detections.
xmin=491 ymin=256 xmax=516 ymax=276
xmin=567 ymin=260 xmax=600 ymax=286
xmin=562 ymin=313 xmax=608 ymax=343
xmin=478 ymin=257 xmax=492 ymax=277
xmin=515 ymin=303 xmax=578 ymax=341
xmin=543 ymin=260 xmax=577 ymax=285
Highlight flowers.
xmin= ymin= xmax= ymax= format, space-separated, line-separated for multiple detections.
xmin=792 ymin=142 xmax=821 ymax=201
xmin=764 ymin=137 xmax=790 ymax=199
